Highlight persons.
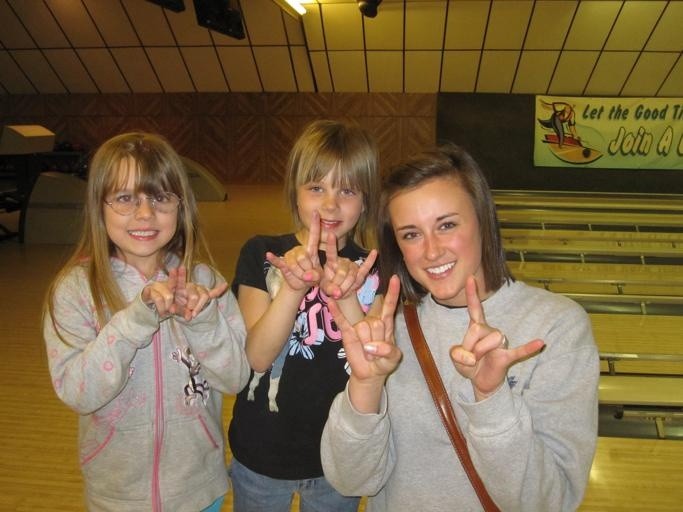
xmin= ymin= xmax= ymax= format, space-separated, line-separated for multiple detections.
xmin=320 ymin=142 xmax=600 ymax=511
xmin=42 ymin=129 xmax=247 ymax=512
xmin=232 ymin=119 xmax=388 ymax=511
xmin=539 ymin=101 xmax=579 ymax=147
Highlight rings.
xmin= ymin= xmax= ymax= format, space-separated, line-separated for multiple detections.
xmin=502 ymin=335 xmax=507 ymax=345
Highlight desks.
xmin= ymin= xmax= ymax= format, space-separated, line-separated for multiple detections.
xmin=0 ymin=150 xmax=60 ymax=244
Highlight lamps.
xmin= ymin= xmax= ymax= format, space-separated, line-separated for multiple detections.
xmin=272 ymin=0 xmax=307 ymax=19
xmin=357 ymin=0 xmax=381 ymax=18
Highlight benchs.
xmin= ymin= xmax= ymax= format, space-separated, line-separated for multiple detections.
xmin=493 ymin=192 xmax=682 ymax=406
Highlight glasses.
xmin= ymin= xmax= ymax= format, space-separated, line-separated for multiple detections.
xmin=102 ymin=192 xmax=183 ymax=216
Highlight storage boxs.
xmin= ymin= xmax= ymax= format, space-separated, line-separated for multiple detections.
xmin=0 ymin=122 xmax=57 ymax=155
